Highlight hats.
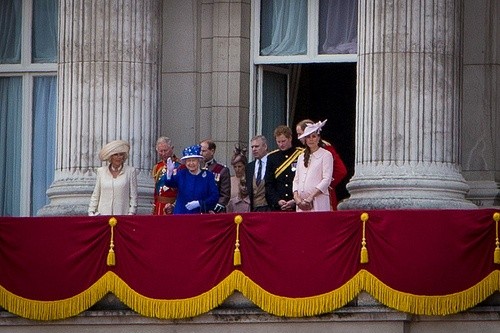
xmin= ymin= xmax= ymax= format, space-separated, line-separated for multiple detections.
xmin=180 ymin=144 xmax=205 ymax=161
xmin=99 ymin=140 xmax=130 ymax=161
xmin=231 ymin=143 xmax=247 ymax=165
xmin=298 ymin=119 xmax=327 ymax=139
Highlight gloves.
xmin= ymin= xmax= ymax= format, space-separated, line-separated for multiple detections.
xmin=167 ymin=157 xmax=175 ymax=179
xmin=88 ymin=212 xmax=94 ymax=216
xmin=129 ymin=213 xmax=134 ymax=215
xmin=185 ymin=200 xmax=200 ymax=210
xmin=213 ymin=203 xmax=226 ymax=213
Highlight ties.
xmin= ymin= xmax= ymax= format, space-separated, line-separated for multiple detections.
xmin=256 ymin=159 xmax=262 ymax=187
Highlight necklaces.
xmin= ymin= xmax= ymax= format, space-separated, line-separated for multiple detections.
xmin=112 ymin=164 xmax=122 ymax=171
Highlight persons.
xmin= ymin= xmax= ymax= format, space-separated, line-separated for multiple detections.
xmin=150 ymin=136 xmax=230 ymax=214
xmin=88 ymin=139 xmax=138 ymax=215
xmin=226 ymin=119 xmax=346 ymax=213
